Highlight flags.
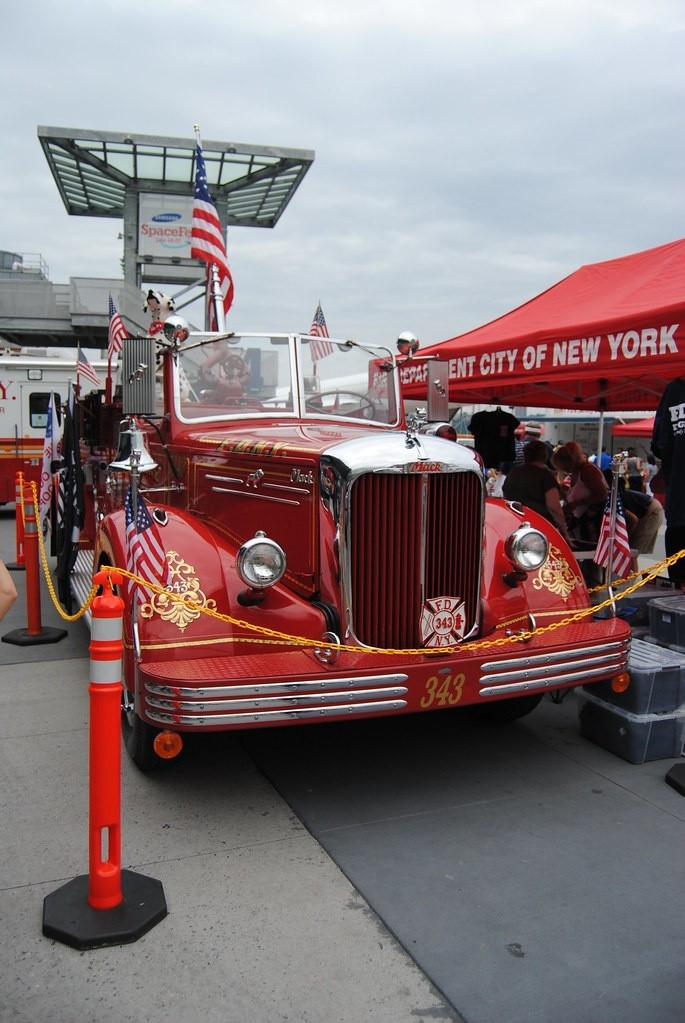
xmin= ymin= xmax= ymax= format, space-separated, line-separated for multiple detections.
xmin=119 ymin=477 xmax=171 ymax=613
xmin=37 ymin=293 xmax=127 ymax=545
xmin=311 ymin=306 xmax=333 ymax=360
xmin=189 ymin=143 xmax=235 ymax=332
xmin=594 ymin=478 xmax=634 ymax=579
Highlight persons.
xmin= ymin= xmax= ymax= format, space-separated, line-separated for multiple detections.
xmin=483 ymin=375 xmax=685 ymax=594
xmin=0 ymin=559 xmax=18 ymax=627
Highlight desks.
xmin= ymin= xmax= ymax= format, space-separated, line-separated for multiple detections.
xmin=572 ymin=548 xmax=638 ymax=597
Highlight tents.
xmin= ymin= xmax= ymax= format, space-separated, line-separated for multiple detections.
xmin=369 ymin=240 xmax=685 ymax=467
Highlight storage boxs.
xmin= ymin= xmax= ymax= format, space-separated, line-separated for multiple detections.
xmin=575 ymin=585 xmax=685 ymax=767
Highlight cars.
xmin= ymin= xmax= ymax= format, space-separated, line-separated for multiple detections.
xmin=48 ymin=316 xmax=636 ymax=779
